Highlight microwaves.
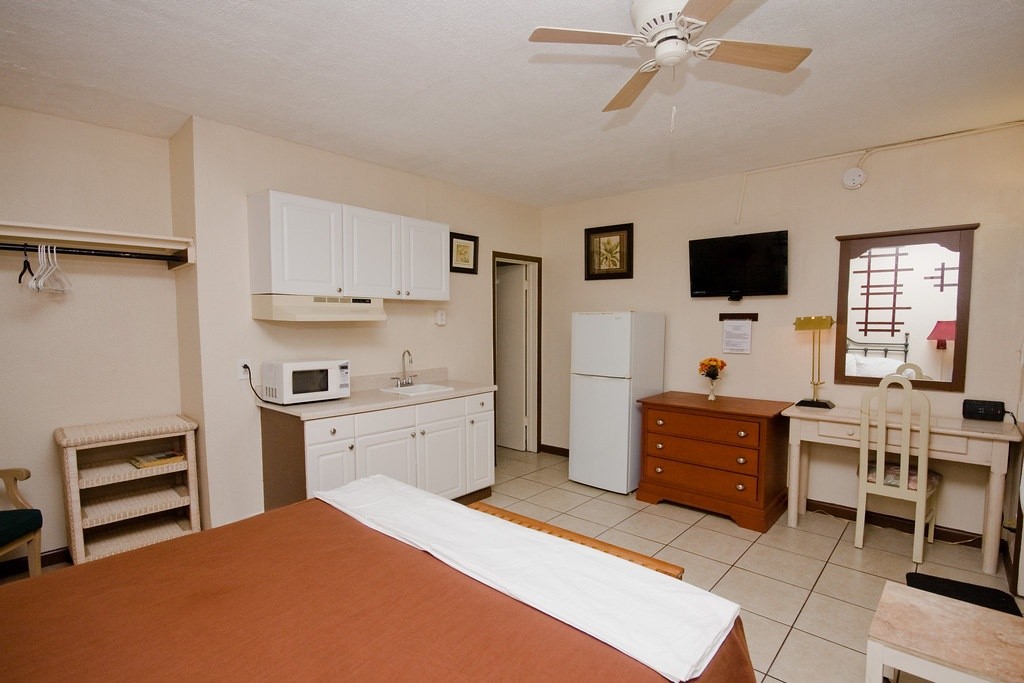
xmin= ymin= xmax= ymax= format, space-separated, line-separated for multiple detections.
xmin=262 ymin=360 xmax=350 ymax=405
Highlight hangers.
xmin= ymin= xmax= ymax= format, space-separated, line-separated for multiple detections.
xmin=18 ymin=243 xmax=74 ymax=297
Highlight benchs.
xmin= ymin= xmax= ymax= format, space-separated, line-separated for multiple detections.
xmin=866 ymin=580 xmax=1024 ymax=683
xmin=467 ymin=502 xmax=685 ymax=581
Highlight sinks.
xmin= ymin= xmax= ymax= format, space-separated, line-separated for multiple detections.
xmin=379 ymin=383 xmax=455 ymax=397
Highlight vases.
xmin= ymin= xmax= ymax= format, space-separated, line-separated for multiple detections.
xmin=708 ymin=380 xmax=716 ymax=401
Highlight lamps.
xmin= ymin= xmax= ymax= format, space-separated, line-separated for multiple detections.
xmin=792 ymin=316 xmax=838 ymax=409
xmin=927 ymin=321 xmax=955 ymax=349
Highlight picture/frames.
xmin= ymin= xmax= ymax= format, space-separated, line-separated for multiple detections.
xmin=450 ymin=231 xmax=479 ymax=274
xmin=584 ymin=223 xmax=634 ymax=281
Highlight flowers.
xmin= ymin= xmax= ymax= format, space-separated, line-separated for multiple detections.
xmin=699 ymin=357 xmax=727 ymax=386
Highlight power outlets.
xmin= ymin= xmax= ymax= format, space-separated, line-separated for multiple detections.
xmin=238 ymin=360 xmax=255 ymax=380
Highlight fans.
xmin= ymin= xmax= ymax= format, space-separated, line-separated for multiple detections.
xmin=528 ymin=0 xmax=813 ymax=133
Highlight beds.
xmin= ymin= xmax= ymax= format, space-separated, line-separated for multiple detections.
xmin=1 ymin=473 xmax=760 ymax=683
xmin=845 ymin=332 xmax=916 ymax=380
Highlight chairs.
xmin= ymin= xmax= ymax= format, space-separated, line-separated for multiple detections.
xmin=0 ymin=468 xmax=43 ymax=579
xmin=854 ymin=376 xmax=943 ymax=564
xmin=886 ymin=364 xmax=934 ymax=380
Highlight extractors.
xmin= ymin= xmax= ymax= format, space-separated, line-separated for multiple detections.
xmin=251 ymin=294 xmax=386 ymax=321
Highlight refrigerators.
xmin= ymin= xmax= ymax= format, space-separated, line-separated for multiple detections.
xmin=568 ymin=312 xmax=665 ymax=494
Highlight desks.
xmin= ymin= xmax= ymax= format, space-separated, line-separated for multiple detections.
xmin=781 ymin=402 xmax=1022 ymax=574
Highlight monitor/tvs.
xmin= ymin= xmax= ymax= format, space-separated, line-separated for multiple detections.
xmin=689 ymin=230 xmax=789 ymax=301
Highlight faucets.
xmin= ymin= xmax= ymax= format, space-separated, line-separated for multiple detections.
xmin=401 ymin=349 xmax=413 ymax=384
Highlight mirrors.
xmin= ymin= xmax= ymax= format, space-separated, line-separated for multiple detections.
xmin=834 ymin=223 xmax=980 ymax=393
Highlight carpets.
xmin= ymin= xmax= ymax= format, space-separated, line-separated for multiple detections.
xmin=906 ymin=572 xmax=1023 ymax=617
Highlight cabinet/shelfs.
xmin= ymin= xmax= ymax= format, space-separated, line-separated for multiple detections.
xmin=54 ymin=414 xmax=198 ymax=567
xmin=636 ymin=390 xmax=795 ymax=534
xmin=260 ymin=407 xmax=355 ymax=511
xmin=465 ymin=392 xmax=495 ymax=505
xmin=343 ymin=204 xmax=450 ymax=301
xmin=247 ymin=189 xmax=344 ymax=296
xmin=354 ymin=397 xmax=467 ymax=516
